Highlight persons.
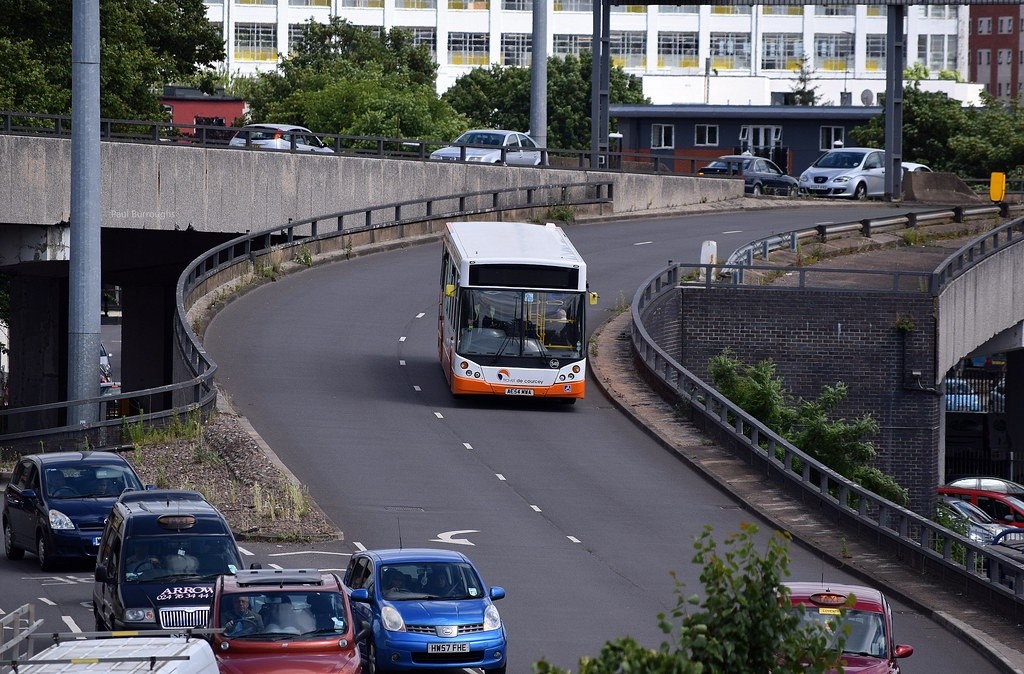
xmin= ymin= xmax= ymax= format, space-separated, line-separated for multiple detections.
xmin=467 ymin=294 xmax=579 ymax=351
xmin=354 ymin=569 xmax=457 ymax=596
xmin=223 ymin=597 xmax=265 ymax=634
xmin=88 ymin=479 xmax=114 ymax=496
xmin=127 ymin=545 xmax=158 ymax=574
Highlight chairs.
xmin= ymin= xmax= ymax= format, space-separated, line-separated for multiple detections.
xmin=301 ymin=594 xmax=335 ymax=631
xmin=82 ymin=473 xmax=114 ymax=495
xmin=401 ymin=571 xmax=438 ymax=593
xmin=842 ymin=615 xmax=882 ymax=654
xmin=164 ymin=552 xmax=200 ymax=575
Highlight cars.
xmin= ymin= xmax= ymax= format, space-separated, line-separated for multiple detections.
xmin=695 ymin=150 xmax=800 ymax=197
xmin=936 ymin=478 xmax=1024 ymax=567
xmin=3 ymin=449 xmax=157 ymax=573
xmin=229 ymin=123 xmax=335 ymax=154
xmin=100 ymin=343 xmax=121 ymax=421
xmin=798 ymin=148 xmax=934 ymax=200
xmin=988 ymin=376 xmax=1005 ymax=412
xmin=945 ymin=379 xmax=982 ymax=412
xmin=737 ymin=582 xmax=914 ymax=674
xmin=430 ymin=129 xmax=549 ymax=167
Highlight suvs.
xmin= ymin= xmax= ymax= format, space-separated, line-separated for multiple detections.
xmin=260 ymin=636 xmax=261 ymax=637
xmin=0 ymin=600 xmax=220 ymax=674
xmin=191 ymin=569 xmax=371 ymax=674
xmin=334 ymin=548 xmax=507 ymax=674
xmin=93 ymin=487 xmax=263 ymax=638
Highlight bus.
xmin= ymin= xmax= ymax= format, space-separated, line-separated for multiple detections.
xmin=438 ymin=222 xmax=600 ymax=404
